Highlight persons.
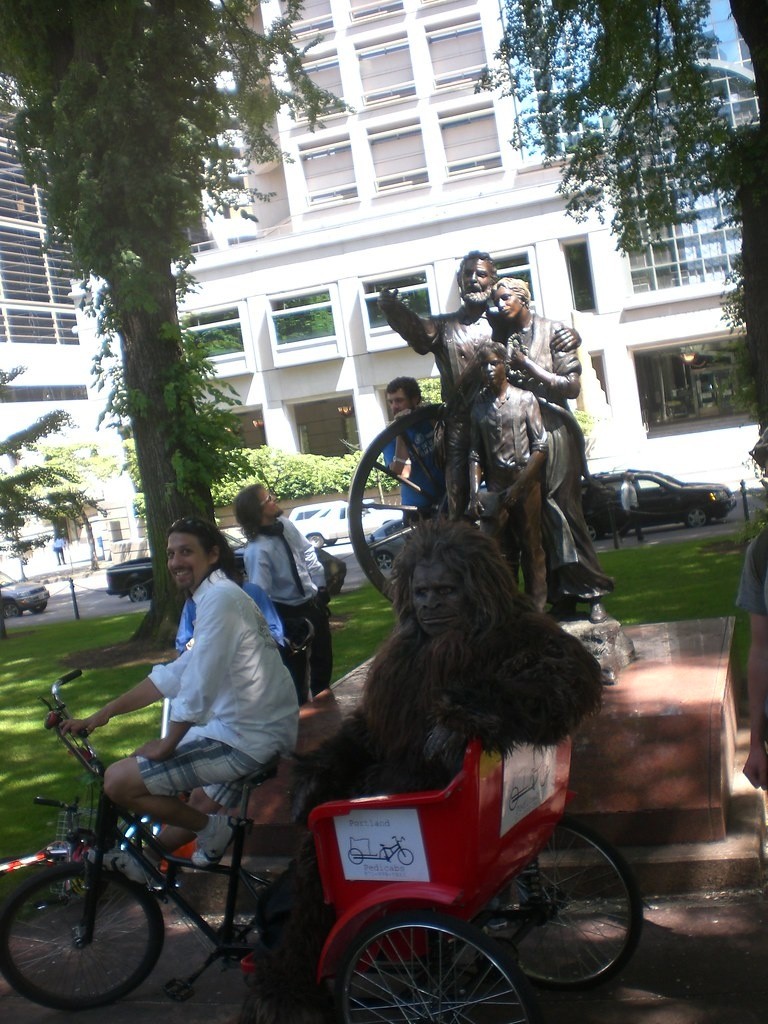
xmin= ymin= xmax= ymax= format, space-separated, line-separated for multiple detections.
xmin=379 ymin=251 xmax=616 ymax=623
xmin=381 ymin=377 xmax=449 ymax=528
xmin=54 ymin=537 xmax=66 ymax=565
xmin=59 ymin=484 xmax=334 ymax=885
xmin=617 ymin=472 xmax=649 ymax=543
xmin=736 ymin=527 xmax=768 ymax=790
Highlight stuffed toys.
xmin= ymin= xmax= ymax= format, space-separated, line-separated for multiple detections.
xmin=289 ymin=521 xmax=603 ymax=877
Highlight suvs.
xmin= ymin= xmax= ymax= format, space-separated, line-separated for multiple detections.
xmin=286 ymin=501 xmax=405 ymax=550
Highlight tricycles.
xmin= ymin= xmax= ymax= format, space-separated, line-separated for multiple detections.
xmin=4 ymin=666 xmax=641 ymax=1024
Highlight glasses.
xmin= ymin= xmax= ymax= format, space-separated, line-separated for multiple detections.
xmin=260 ymin=493 xmax=274 ymax=509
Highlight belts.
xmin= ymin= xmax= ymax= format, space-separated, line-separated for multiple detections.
xmin=273 ymin=595 xmax=319 ymax=609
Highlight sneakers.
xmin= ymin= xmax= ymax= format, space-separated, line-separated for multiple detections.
xmin=87 ymin=850 xmax=162 ymax=886
xmin=191 ymin=814 xmax=238 ymax=869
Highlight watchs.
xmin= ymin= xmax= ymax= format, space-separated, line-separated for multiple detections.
xmin=393 ymin=456 xmax=406 ymax=464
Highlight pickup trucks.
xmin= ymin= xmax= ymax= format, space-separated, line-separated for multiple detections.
xmin=102 ymin=531 xmax=244 ymax=603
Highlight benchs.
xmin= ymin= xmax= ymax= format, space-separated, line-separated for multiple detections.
xmin=305 ymin=727 xmax=572 ymax=894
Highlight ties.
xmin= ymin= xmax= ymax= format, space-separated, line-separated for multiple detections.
xmin=259 ymin=522 xmax=306 ymax=597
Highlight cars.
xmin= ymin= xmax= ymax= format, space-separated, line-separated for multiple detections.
xmin=582 ymin=469 xmax=739 ymax=542
xmin=0 ymin=570 xmax=50 ymax=619
xmin=369 ymin=518 xmax=413 ymax=571
